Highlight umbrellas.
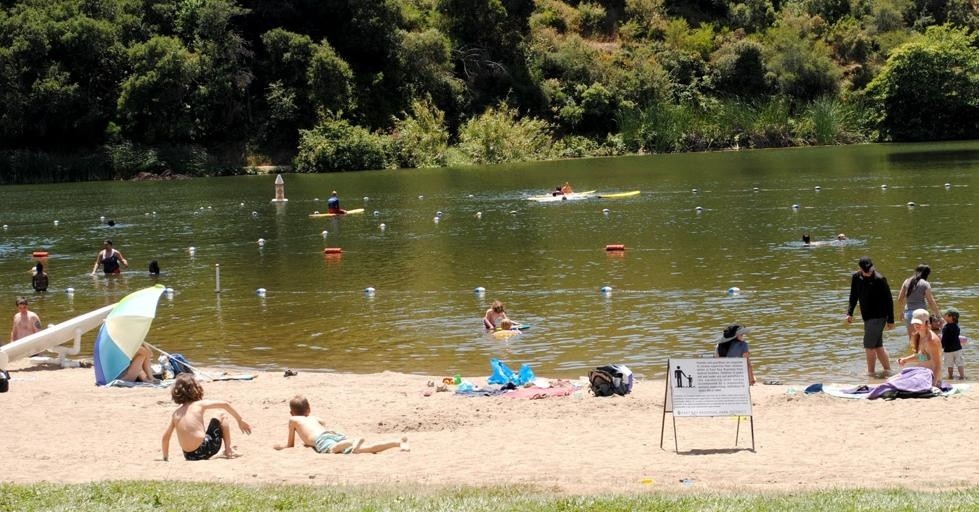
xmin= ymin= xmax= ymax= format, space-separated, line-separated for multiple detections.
xmin=93 ymin=284 xmax=215 ymax=386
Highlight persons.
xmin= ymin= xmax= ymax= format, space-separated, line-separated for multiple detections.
xmin=561 ymin=182 xmax=572 ymax=193
xmin=553 ymin=185 xmax=564 ymax=195
xmin=120 ymin=345 xmax=159 ymax=384
xmin=803 ymin=234 xmax=813 ymax=246
xmin=91 ymin=238 xmax=128 ymax=276
xmin=10 ymin=297 xmax=42 ymax=357
xmin=157 ymin=372 xmax=252 ymax=463
xmin=838 ymin=234 xmax=846 ymax=241
xmin=492 ymin=318 xmax=517 ymax=337
xmin=844 ymin=255 xmax=966 ymax=389
xmin=272 ymin=394 xmax=410 ymax=454
xmin=327 ymin=190 xmax=344 ymax=214
xmin=32 ymin=263 xmax=48 ymax=292
xmin=484 ymin=301 xmax=507 ymax=330
xmin=713 ymin=324 xmax=755 ymax=385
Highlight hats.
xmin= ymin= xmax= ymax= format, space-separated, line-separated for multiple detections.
xmin=911 ymin=309 xmax=929 ymax=325
xmin=716 ymin=325 xmax=748 ymax=344
xmin=859 ymin=258 xmax=872 ymax=272
